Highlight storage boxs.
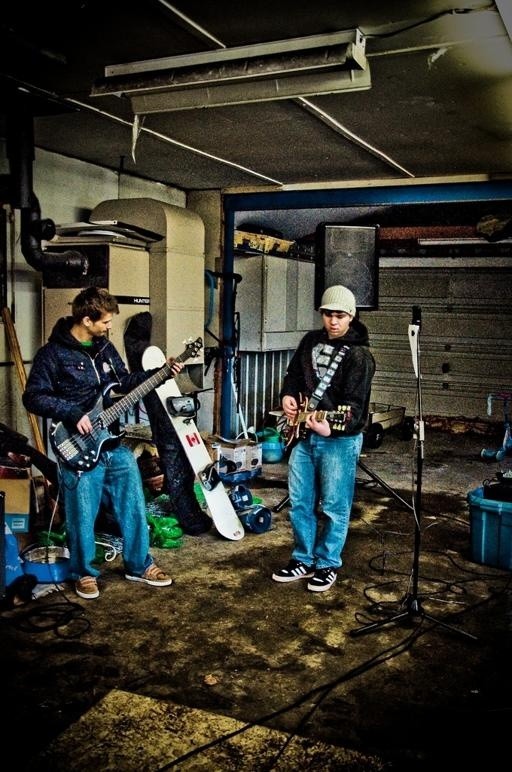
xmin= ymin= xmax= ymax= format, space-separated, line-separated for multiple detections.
xmin=469 ymin=484 xmax=512 ymax=571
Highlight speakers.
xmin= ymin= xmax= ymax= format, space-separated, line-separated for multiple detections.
xmin=315 ymin=221 xmax=379 ymax=311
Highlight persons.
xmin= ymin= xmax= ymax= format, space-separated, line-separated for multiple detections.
xmin=21 ymin=285 xmax=186 ymax=600
xmin=271 ymin=284 xmax=378 ymax=593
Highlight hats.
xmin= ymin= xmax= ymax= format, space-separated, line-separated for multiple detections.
xmin=318 ymin=285 xmax=356 ymax=318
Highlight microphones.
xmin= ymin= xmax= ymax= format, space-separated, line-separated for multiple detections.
xmin=410 ymin=304 xmax=423 ymax=335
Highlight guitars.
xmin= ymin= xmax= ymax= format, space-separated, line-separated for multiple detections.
xmin=49 ymin=337 xmax=203 ymax=472
xmin=284 ymin=392 xmax=352 ymax=448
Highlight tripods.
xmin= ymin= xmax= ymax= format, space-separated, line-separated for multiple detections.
xmin=349 ymin=338 xmax=477 ymax=642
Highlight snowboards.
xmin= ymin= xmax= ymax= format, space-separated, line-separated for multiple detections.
xmin=142 ymin=345 xmax=245 ymax=540
xmin=123 ymin=313 xmax=212 ymax=536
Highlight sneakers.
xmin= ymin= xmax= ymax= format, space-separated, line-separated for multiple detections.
xmin=271 ymin=559 xmax=337 ymax=592
xmin=124 ymin=564 xmax=172 ymax=587
xmin=76 ymin=575 xmax=100 ymax=599
